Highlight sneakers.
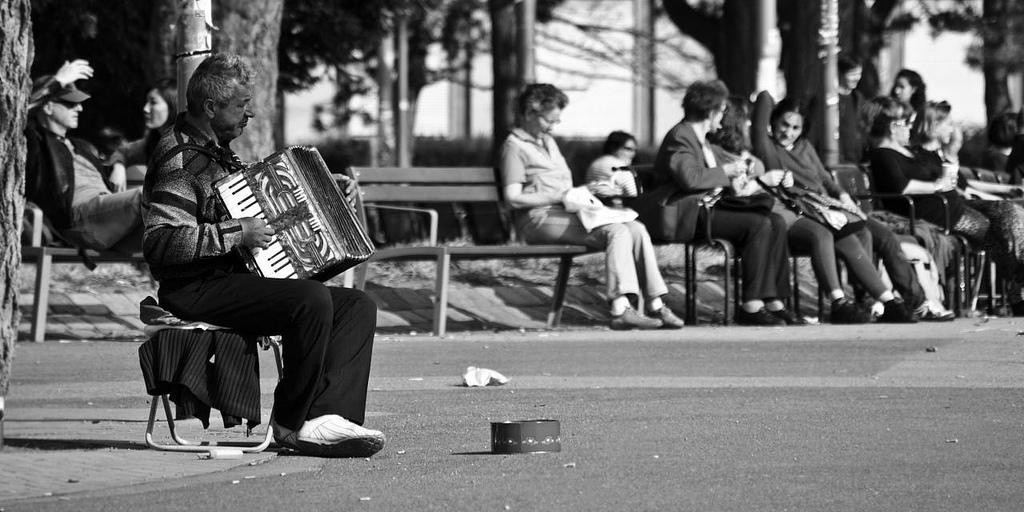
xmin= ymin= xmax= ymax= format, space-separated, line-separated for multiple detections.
xmin=649 ymin=306 xmax=685 ymax=329
xmin=734 ymin=305 xmax=786 ymax=326
xmin=883 ymin=298 xmax=919 ymax=323
xmin=274 ymin=414 xmax=387 ymax=458
xmin=767 ymin=307 xmax=809 ymax=326
xmin=916 ymin=301 xmax=956 ymax=322
xmin=830 ymin=297 xmax=871 ymax=324
xmin=609 ymin=306 xmax=664 ymax=331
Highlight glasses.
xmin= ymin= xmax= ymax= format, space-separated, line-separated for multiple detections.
xmin=892 ymin=118 xmax=910 ymax=126
xmin=540 ymin=115 xmax=560 ymax=125
xmin=623 ymin=146 xmax=638 ymax=152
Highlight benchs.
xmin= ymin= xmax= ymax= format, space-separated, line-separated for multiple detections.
xmin=344 ymin=166 xmax=604 ymax=338
xmin=21 ymin=165 xmax=145 ymax=342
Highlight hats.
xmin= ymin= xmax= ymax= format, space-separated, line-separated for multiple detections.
xmin=33 ymin=75 xmax=91 ymax=103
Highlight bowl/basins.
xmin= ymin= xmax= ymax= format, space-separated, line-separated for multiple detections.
xmin=488 ymin=418 xmax=562 ymax=453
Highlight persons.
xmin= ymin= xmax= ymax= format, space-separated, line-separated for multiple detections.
xmin=910 ymin=100 xmax=967 ymax=200
xmin=100 ymin=78 xmax=177 ymax=194
xmin=967 ymin=107 xmax=1023 ymax=199
xmin=749 ymin=88 xmax=957 ymax=322
xmin=644 ymin=78 xmax=808 ymax=326
xmin=704 ymin=95 xmax=920 ymax=323
xmin=585 ymin=132 xmax=643 ymax=205
xmin=498 ymin=82 xmax=686 ymax=330
xmin=891 ymin=69 xmax=926 ymax=139
xmin=23 ymin=58 xmax=144 ymax=255
xmin=859 ymin=98 xmax=1024 ymax=315
xmin=806 ymin=52 xmax=869 ymax=162
xmin=140 ymin=53 xmax=388 ymax=454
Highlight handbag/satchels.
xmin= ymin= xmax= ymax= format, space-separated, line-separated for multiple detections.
xmin=793 ymin=192 xmax=867 ymax=242
xmin=716 ymin=194 xmax=775 ymax=211
xmin=562 ymin=184 xmax=640 ymax=235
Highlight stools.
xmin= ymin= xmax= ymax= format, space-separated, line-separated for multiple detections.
xmin=140 ymin=321 xmax=283 ymax=454
